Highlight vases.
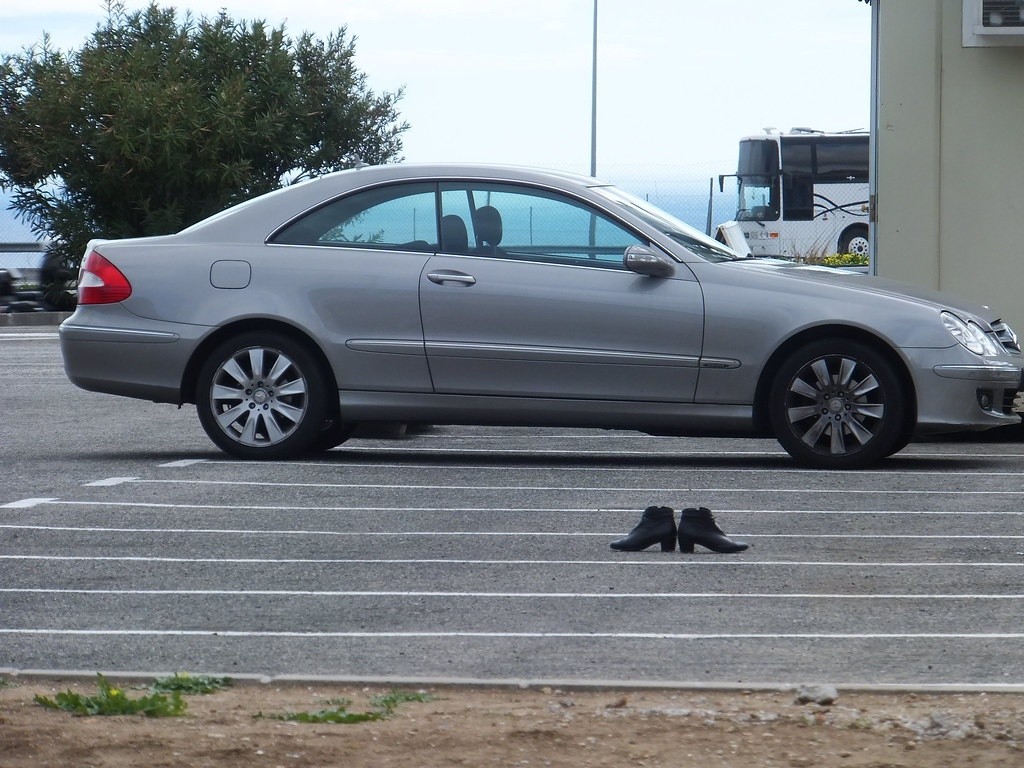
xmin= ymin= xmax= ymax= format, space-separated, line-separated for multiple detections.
xmin=836 ymin=266 xmax=869 ymax=273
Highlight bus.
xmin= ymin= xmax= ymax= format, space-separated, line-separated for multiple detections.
xmin=719 ymin=126 xmax=870 ymax=258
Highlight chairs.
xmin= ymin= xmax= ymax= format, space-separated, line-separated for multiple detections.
xmin=476 ymin=205 xmax=503 ymax=255
xmin=441 ymin=215 xmax=470 ymax=254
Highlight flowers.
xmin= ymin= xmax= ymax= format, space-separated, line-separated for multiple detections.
xmin=824 ymin=254 xmax=869 ymax=265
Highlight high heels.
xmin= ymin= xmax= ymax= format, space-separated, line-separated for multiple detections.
xmin=677 ymin=507 xmax=749 ymax=553
xmin=610 ymin=505 xmax=678 ymax=552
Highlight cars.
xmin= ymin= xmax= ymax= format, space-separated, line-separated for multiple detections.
xmin=57 ymin=163 xmax=1024 ymax=471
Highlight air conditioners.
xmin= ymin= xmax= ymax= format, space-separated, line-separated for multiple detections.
xmin=973 ymin=0 xmax=1024 ymax=35
